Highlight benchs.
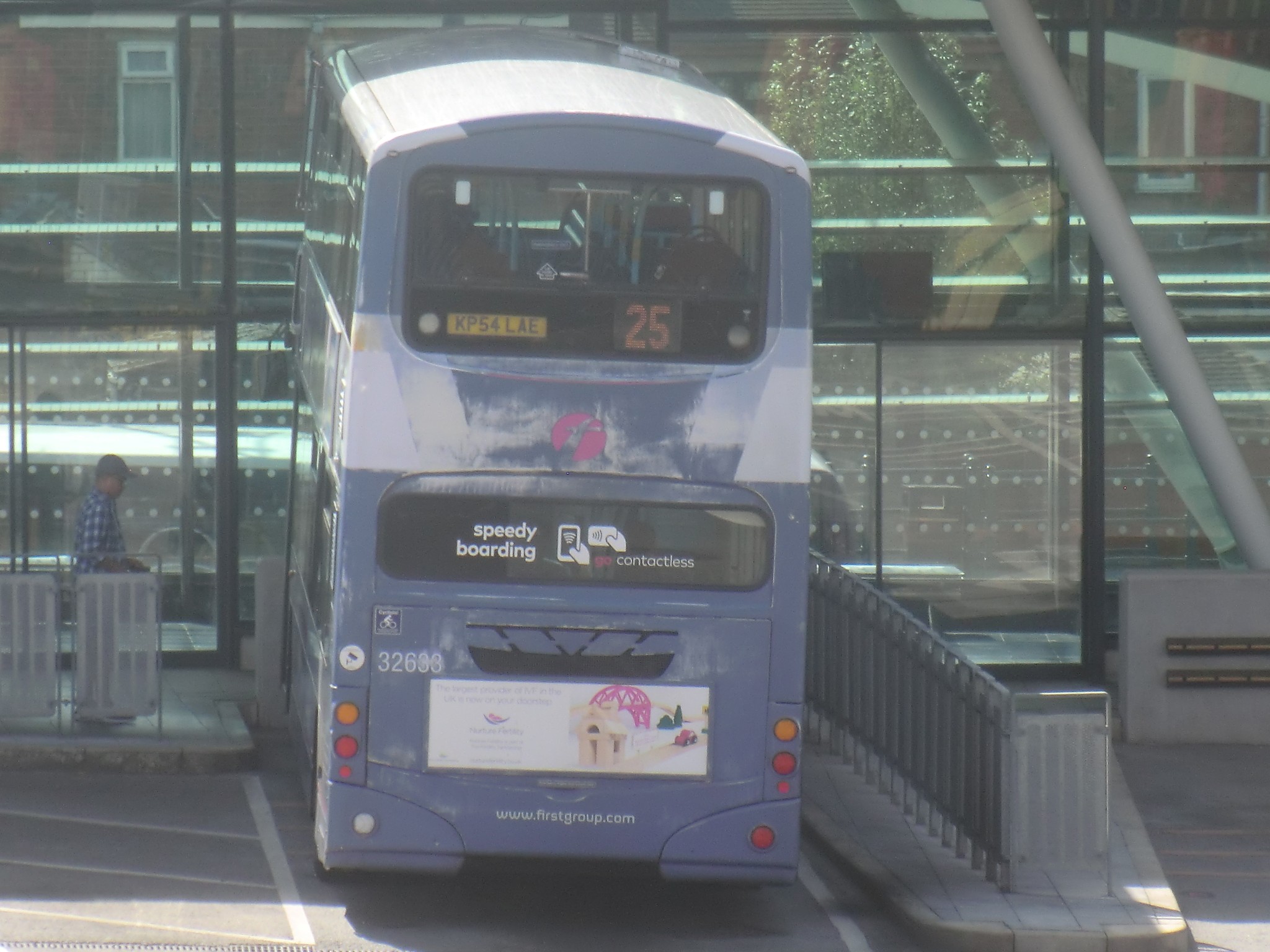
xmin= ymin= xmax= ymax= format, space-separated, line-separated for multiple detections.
xmin=843 ymin=565 xmax=965 ymax=630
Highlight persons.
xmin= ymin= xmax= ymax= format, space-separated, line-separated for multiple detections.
xmin=74 ymin=454 xmax=151 ymax=723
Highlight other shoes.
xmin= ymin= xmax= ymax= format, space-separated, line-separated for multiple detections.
xmin=105 ymin=716 xmax=136 ymax=724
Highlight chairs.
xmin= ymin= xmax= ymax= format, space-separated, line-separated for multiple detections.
xmin=637 ymin=184 xmax=744 ymax=295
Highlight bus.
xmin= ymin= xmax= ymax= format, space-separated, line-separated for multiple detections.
xmin=253 ymin=26 xmax=817 ymax=885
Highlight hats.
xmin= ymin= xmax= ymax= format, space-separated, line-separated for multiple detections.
xmin=96 ymin=454 xmax=137 ymax=478
